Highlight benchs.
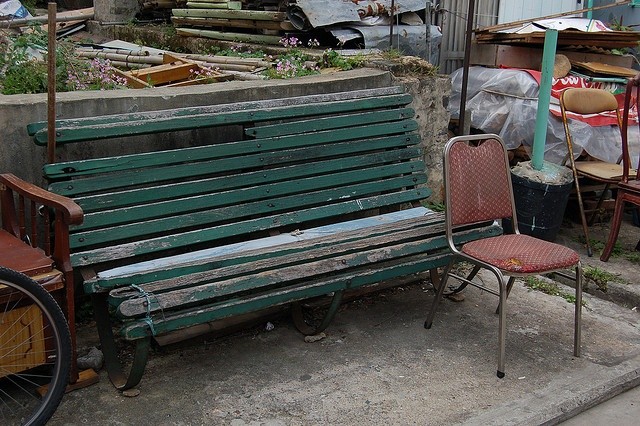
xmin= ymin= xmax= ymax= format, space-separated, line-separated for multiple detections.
xmin=25 ymin=85 xmax=503 ymax=395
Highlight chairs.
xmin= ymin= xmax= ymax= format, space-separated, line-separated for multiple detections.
xmin=598 ymin=80 xmax=640 ymax=264
xmin=422 ymin=131 xmax=583 ymax=377
xmin=557 ymin=85 xmax=638 ymax=257
xmin=0 ymin=172 xmax=84 ymax=377
